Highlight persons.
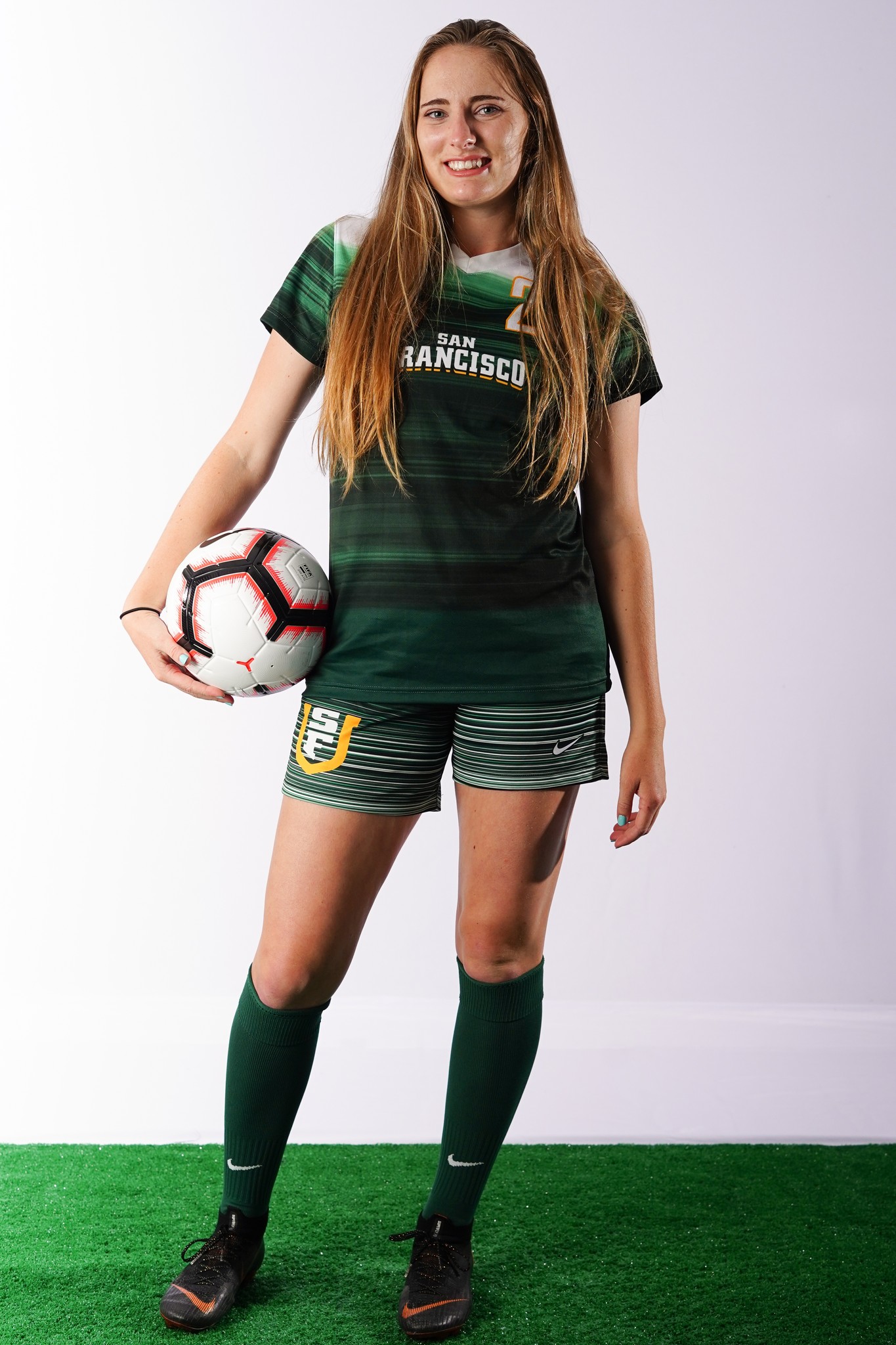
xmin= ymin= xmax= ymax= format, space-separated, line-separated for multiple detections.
xmin=117 ymin=20 xmax=670 ymax=1340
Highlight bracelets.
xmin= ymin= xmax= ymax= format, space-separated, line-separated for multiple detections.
xmin=120 ymin=607 xmax=160 ymax=620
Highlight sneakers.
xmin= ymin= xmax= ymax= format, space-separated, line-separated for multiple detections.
xmin=159 ymin=1205 xmax=265 ymax=1331
xmin=387 ymin=1209 xmax=475 ymax=1340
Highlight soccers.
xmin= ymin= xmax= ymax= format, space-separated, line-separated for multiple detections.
xmin=164 ymin=526 xmax=331 ymax=695
